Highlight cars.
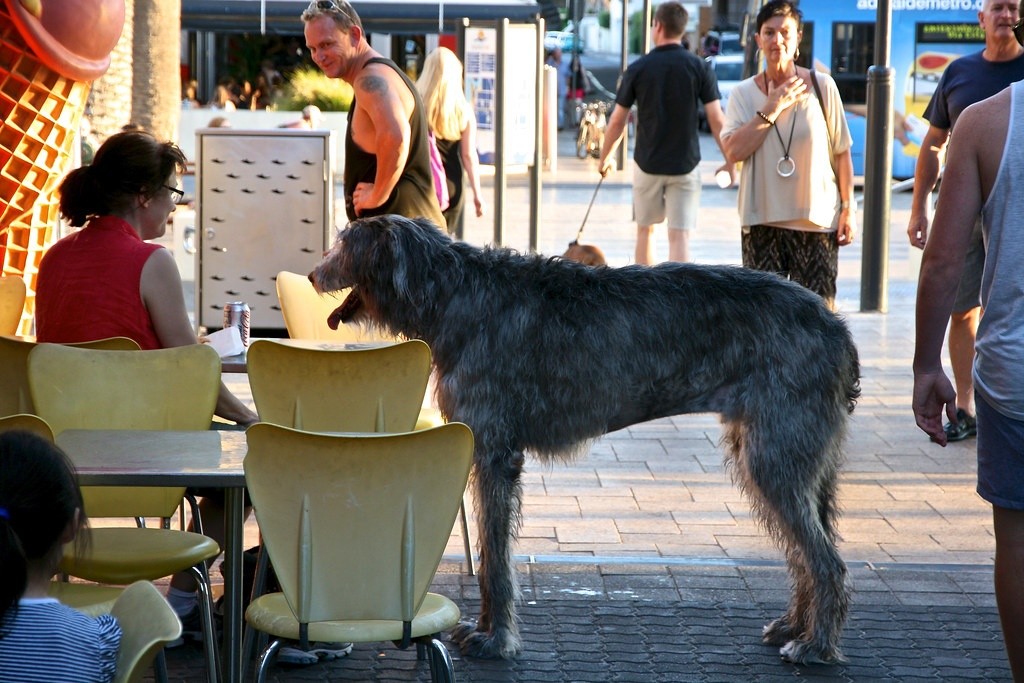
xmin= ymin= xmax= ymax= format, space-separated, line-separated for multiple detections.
xmin=544 ymin=29 xmax=583 ymax=54
xmin=698 ymin=54 xmax=745 ymax=135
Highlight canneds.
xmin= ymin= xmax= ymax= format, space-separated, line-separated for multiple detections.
xmin=223 ymin=301 xmax=250 ymax=348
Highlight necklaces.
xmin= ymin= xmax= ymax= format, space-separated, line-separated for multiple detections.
xmin=764 ymin=64 xmax=798 ymax=177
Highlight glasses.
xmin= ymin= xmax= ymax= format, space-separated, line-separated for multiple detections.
xmin=162 ymin=184 xmax=184 ymax=204
xmin=1012 ymin=18 xmax=1024 ymax=48
xmin=317 ymin=0 xmax=356 ymax=26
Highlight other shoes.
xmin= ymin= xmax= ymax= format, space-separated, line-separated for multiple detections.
xmin=930 ymin=407 xmax=977 ymax=442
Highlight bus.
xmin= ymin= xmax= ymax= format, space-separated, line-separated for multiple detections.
xmin=756 ymin=0 xmax=987 ymax=191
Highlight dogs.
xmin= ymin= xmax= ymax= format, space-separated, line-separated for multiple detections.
xmin=562 ymin=243 xmax=607 ymax=267
xmin=312 ymin=215 xmax=866 ymax=666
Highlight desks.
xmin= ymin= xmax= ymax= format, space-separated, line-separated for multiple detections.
xmin=55 ymin=425 xmax=396 ymax=683
xmin=221 ymin=337 xmax=400 ymax=374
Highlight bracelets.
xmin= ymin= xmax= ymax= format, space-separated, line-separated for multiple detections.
xmin=756 ymin=111 xmax=774 ymax=126
xmin=843 ymin=200 xmax=858 ymax=212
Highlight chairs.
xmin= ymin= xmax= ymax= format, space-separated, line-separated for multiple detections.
xmin=0 ymin=269 xmax=476 ymax=683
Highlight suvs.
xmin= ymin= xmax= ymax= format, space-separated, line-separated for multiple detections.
xmin=703 ymin=28 xmax=743 ymax=57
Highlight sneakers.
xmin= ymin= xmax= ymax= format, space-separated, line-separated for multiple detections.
xmin=278 ymin=624 xmax=354 ymax=665
xmin=165 ymin=603 xmax=224 ymax=649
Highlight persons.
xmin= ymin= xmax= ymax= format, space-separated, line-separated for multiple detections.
xmin=911 ymin=76 xmax=1024 ymax=683
xmin=300 ymin=0 xmax=451 ymax=245
xmin=720 ymin=1 xmax=858 ymax=317
xmin=566 ymin=57 xmax=589 ymax=128
xmin=35 ymin=131 xmax=355 ymax=664
xmin=597 ymin=3 xmax=737 ymax=267
xmin=182 ymin=70 xmax=274 ymax=112
xmin=412 ymin=46 xmax=484 ymax=245
xmin=907 ymin=0 xmax=1024 ymax=442
xmin=546 ymin=46 xmax=572 ymax=131
xmin=0 ymin=429 xmax=123 ymax=683
